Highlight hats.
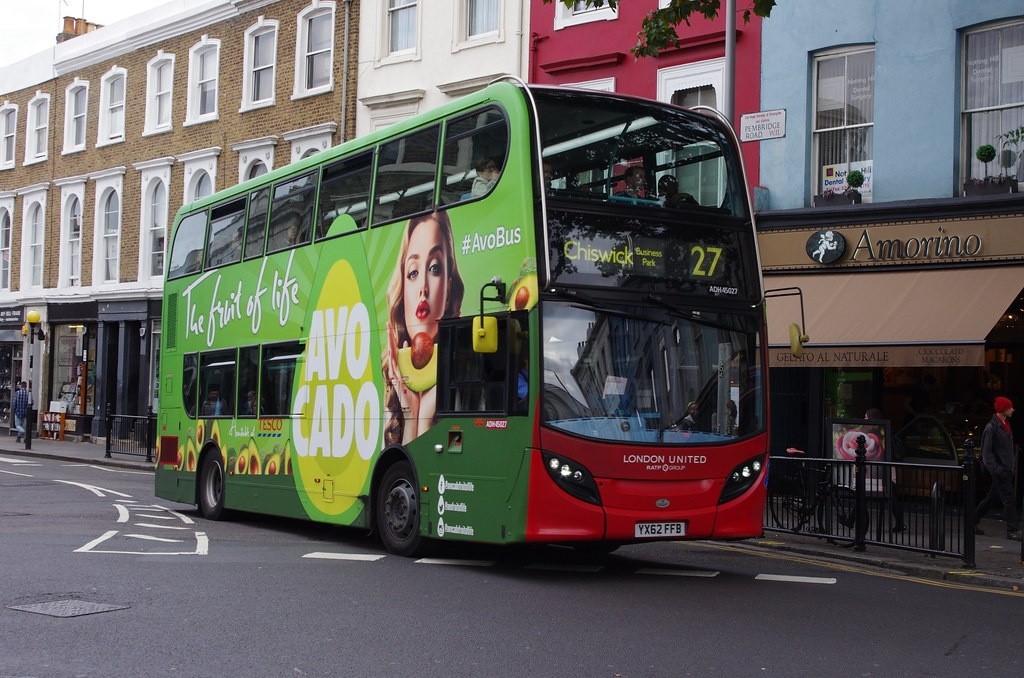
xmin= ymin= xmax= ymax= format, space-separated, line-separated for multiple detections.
xmin=687 ymin=400 xmax=699 ymax=409
xmin=994 ymin=397 xmax=1012 ymax=413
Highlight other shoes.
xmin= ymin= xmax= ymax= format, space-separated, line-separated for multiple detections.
xmin=837 ymin=516 xmax=854 ymax=529
xmin=15 ymin=437 xmax=21 ymax=443
xmin=888 ymin=525 xmax=906 ymax=533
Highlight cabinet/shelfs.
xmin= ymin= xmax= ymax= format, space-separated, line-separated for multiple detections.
xmin=39 ymin=411 xmax=65 ymax=441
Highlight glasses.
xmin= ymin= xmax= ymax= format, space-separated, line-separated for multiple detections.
xmin=486 ymin=165 xmax=497 ymax=170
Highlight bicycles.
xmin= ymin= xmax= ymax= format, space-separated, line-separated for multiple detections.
xmin=766 ymin=446 xmax=870 ymax=546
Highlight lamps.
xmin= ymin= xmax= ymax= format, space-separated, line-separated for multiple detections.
xmin=22 ymin=310 xmax=46 ymax=344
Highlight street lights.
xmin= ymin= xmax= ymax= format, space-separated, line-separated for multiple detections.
xmin=21 ymin=310 xmax=45 ymax=451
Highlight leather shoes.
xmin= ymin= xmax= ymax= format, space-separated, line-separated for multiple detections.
xmin=976 ymin=528 xmax=985 ymax=535
xmin=1007 ymin=532 xmax=1022 ymax=541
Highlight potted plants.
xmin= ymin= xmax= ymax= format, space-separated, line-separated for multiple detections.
xmin=963 ymin=144 xmax=1017 ymax=197
xmin=813 ymin=170 xmax=865 ymax=206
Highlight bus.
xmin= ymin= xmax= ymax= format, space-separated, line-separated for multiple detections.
xmin=154 ymin=75 xmax=770 ymax=557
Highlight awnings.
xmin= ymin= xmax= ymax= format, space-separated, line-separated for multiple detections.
xmin=730 ymin=265 xmax=1024 ymax=372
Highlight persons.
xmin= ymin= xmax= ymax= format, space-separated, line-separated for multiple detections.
xmin=513 ymin=352 xmax=532 ymax=404
xmin=382 ymin=208 xmax=466 ymax=447
xmin=973 ymin=395 xmax=1024 ymax=540
xmin=679 ymin=401 xmax=700 ymax=432
xmin=281 ymin=153 xmax=700 ymax=246
xmin=839 ymin=408 xmax=908 ymax=531
xmin=12 ymin=380 xmax=34 ymax=444
xmin=189 ymin=388 xmax=268 ymax=416
